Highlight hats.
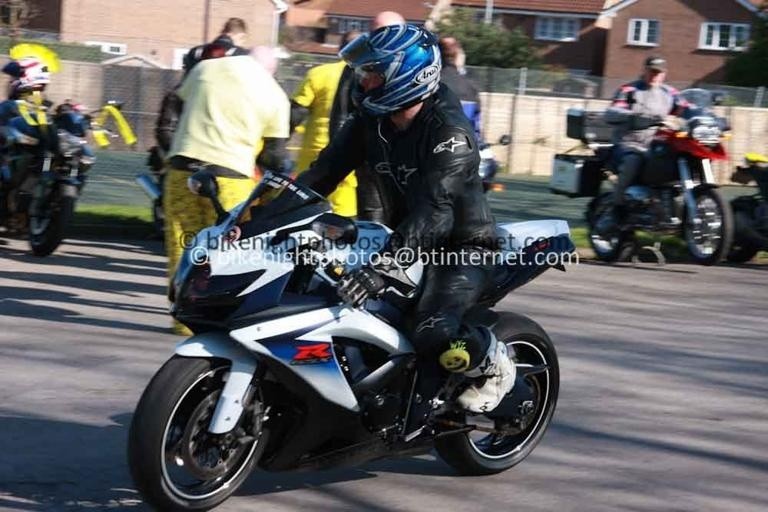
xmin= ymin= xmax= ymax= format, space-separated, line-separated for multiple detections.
xmin=644 ymin=56 xmax=667 ymax=72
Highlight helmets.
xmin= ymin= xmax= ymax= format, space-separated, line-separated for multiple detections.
xmin=339 ymin=24 xmax=442 ymax=119
xmin=1 ymin=57 xmax=50 ymax=99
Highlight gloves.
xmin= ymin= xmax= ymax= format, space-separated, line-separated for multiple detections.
xmin=629 ymin=113 xmax=652 ymax=130
xmin=336 ymin=267 xmax=387 ymax=309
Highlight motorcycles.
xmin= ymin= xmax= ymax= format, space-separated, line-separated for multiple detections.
xmin=549 ymin=85 xmax=737 ymax=267
xmin=1 ymin=90 xmax=126 ymax=258
xmin=724 ymin=155 xmax=768 ymax=266
xmin=132 ymin=153 xmax=301 ymax=251
xmin=476 ymin=136 xmax=513 ymax=191
xmin=128 ymin=162 xmax=576 ymax=511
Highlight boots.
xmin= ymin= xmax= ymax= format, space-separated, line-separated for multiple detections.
xmin=456 ymin=324 xmax=517 ymax=414
xmin=593 ymin=203 xmax=624 ymax=235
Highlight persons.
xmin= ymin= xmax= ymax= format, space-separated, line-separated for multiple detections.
xmin=290 ymin=28 xmax=365 ymax=224
xmin=226 ymin=22 xmax=521 ymax=414
xmin=436 ymin=36 xmax=482 ymax=148
xmin=180 ymin=18 xmax=254 ymax=66
xmin=606 ymin=54 xmax=730 ymax=209
xmin=328 ymin=10 xmax=408 ymax=225
xmin=163 ymin=42 xmax=294 ymax=337
xmin=156 ymin=82 xmax=185 ymax=157
xmin=0 ymin=55 xmax=97 ymax=221
